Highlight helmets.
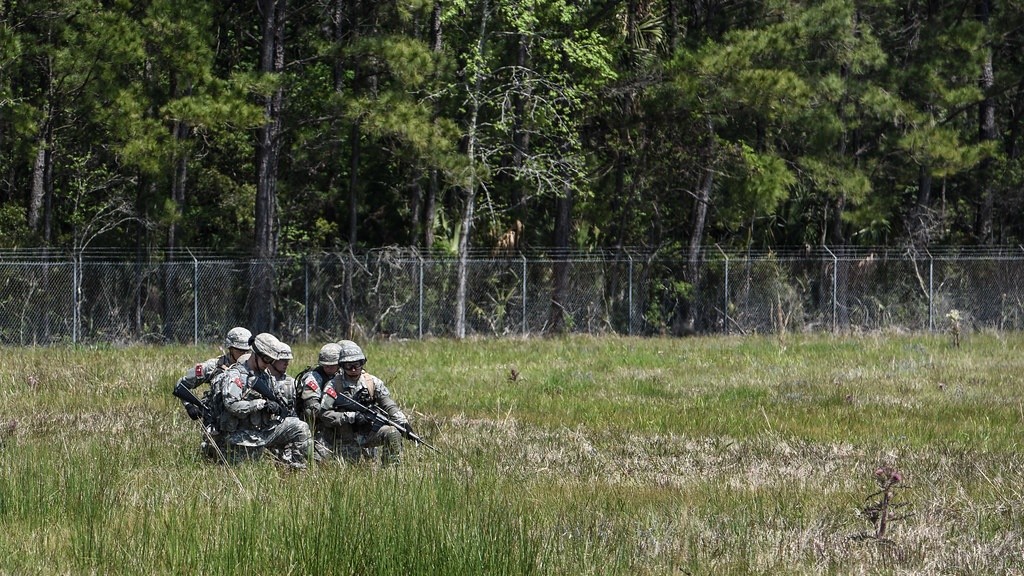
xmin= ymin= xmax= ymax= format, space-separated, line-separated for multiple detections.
xmin=251 ymin=332 xmax=281 ymax=361
xmin=279 ymin=342 xmax=294 ymax=360
xmin=338 ymin=345 xmax=365 ymax=363
xmin=226 ymin=326 xmax=253 ymax=351
xmin=317 ymin=343 xmax=343 ymax=366
xmin=337 ymin=339 xmax=358 ymax=348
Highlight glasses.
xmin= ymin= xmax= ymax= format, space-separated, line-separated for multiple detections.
xmin=261 ymin=354 xmax=275 ymax=364
xmin=339 ymin=361 xmax=364 ymax=370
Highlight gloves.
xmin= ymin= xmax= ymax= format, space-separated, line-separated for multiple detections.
xmin=262 ymin=397 xmax=283 ymax=415
xmin=355 ymin=413 xmax=369 ymax=426
xmin=185 ymin=403 xmax=202 ymax=421
xmin=399 ymin=423 xmax=415 ymax=442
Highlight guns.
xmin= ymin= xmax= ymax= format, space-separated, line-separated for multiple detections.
xmin=336 ymin=392 xmax=449 ymax=457
xmin=172 ymin=382 xmax=217 ymax=425
xmin=252 ymin=377 xmax=290 ymax=423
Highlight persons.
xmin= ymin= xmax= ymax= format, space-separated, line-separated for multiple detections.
xmin=221 ymin=333 xmax=312 ymax=471
xmin=297 ymin=340 xmax=420 ymax=469
xmin=177 ymin=327 xmax=251 ymax=465
